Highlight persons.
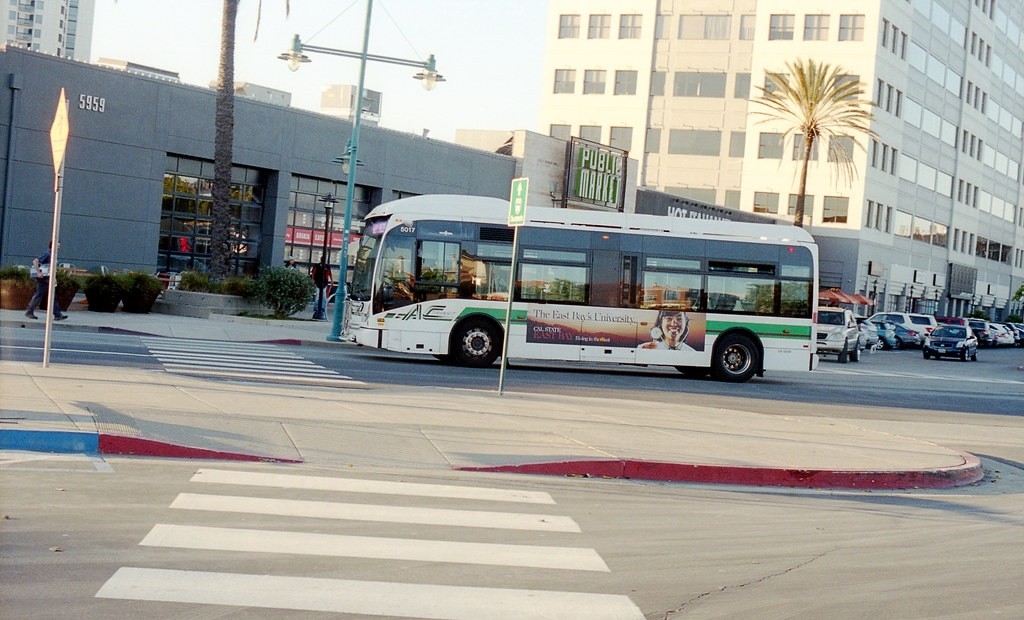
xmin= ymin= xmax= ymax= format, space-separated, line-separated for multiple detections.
xmin=25 ymin=240 xmax=69 ymax=321
xmin=285 ymin=256 xmax=296 ymax=270
xmin=637 ymin=310 xmax=696 ymax=352
xmin=313 ymin=256 xmax=333 ymax=317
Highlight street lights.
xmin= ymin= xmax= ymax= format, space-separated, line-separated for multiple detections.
xmin=279 ymin=36 xmax=447 ymax=340
xmin=313 ymin=191 xmax=340 ymax=321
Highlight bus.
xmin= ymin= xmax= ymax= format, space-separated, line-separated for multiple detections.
xmin=344 ymin=193 xmax=819 ymax=383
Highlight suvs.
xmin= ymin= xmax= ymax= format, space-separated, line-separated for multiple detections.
xmin=854 ymin=314 xmax=878 ymax=350
xmin=866 ymin=313 xmax=937 ymax=349
xmin=969 ymin=318 xmax=989 ymax=345
xmin=934 ymin=316 xmax=964 ymax=326
xmin=815 ymin=305 xmax=862 ymax=364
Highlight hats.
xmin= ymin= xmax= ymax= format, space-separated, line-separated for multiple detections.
xmin=288 ymin=255 xmax=293 ymax=261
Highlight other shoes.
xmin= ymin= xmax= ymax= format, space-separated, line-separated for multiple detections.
xmin=25 ymin=310 xmax=38 ymax=319
xmin=54 ymin=315 xmax=68 ymax=321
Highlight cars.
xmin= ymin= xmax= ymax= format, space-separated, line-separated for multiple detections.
xmin=922 ymin=324 xmax=978 ymax=362
xmin=872 ymin=320 xmax=896 ymax=350
xmin=989 ymin=322 xmax=1024 ymax=348
xmin=160 ymin=218 xmax=245 ymax=256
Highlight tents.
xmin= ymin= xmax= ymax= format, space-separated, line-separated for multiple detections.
xmin=819 ymin=288 xmax=873 ymax=316
